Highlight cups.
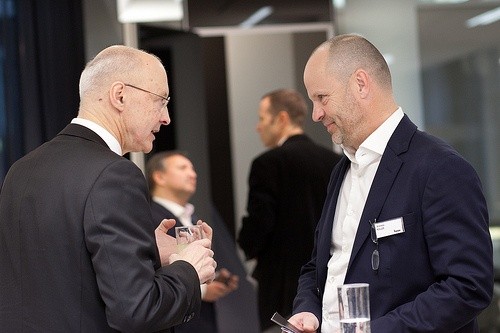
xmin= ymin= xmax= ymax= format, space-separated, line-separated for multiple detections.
xmin=337 ymin=283 xmax=371 ymax=333
xmin=175 ymin=225 xmax=203 ymax=255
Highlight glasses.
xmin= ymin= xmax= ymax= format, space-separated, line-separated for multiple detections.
xmin=125 ymin=83 xmax=170 ymax=108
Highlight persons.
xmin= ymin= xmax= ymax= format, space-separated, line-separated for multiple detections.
xmin=237 ymin=88 xmax=344 ymax=333
xmin=280 ymin=33 xmax=494 ymax=333
xmin=145 ymin=151 xmax=239 ymax=333
xmin=0 ymin=45 xmax=218 ymax=333
